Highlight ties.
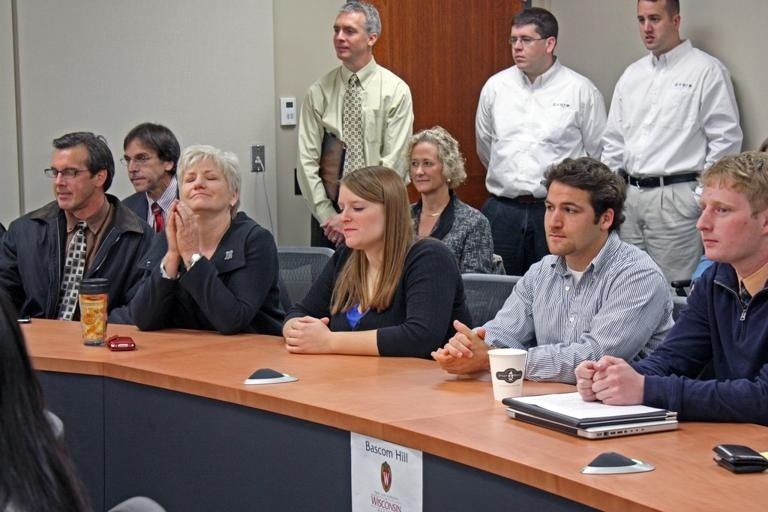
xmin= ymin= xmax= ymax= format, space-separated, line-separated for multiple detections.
xmin=57 ymin=221 xmax=87 ymax=321
xmin=341 ymin=74 xmax=366 ymax=177
xmin=151 ymin=201 xmax=166 ymax=233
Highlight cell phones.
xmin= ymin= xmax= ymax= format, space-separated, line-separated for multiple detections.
xmin=107 ymin=336 xmax=136 ymax=352
xmin=15 ymin=314 xmax=31 ymax=323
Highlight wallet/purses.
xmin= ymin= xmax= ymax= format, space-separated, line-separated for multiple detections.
xmin=712 ymin=445 xmax=768 ymax=474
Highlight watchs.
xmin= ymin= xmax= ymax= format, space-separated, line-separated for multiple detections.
xmin=185 ymin=252 xmax=203 ymax=271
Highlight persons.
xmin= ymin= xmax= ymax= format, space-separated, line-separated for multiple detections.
xmin=0 ymin=290 xmax=89 ymax=511
xmin=475 ymin=7 xmax=607 ymax=277
xmin=120 ymin=122 xmax=182 ymax=233
xmin=296 ymin=0 xmax=415 ymax=252
xmin=128 ymin=145 xmax=292 ymax=337
xmin=573 ymin=150 xmax=768 ymax=425
xmin=407 ymin=126 xmax=493 ymax=274
xmin=430 ymin=157 xmax=674 ymax=384
xmin=600 ymin=0 xmax=744 ymax=290
xmin=281 ymin=166 xmax=473 ymax=359
xmin=0 ymin=131 xmax=168 ymax=324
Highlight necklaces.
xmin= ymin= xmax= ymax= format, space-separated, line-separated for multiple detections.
xmin=421 ymin=210 xmax=442 ymax=216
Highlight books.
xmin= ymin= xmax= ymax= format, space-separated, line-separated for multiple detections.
xmin=501 ymin=392 xmax=678 ymax=429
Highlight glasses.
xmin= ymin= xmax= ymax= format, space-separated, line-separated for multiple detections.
xmin=509 ymin=35 xmax=544 ymax=45
xmin=120 ymin=155 xmax=160 ymax=165
xmin=43 ymin=167 xmax=92 ymax=180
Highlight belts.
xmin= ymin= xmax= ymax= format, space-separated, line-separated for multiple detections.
xmin=623 ymin=171 xmax=699 ymax=188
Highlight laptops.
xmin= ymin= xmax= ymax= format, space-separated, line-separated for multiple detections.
xmin=509 ymin=406 xmax=678 ymax=440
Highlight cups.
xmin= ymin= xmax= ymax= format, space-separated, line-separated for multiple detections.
xmin=79 ymin=278 xmax=110 ymax=345
xmin=487 ymin=348 xmax=527 ymax=402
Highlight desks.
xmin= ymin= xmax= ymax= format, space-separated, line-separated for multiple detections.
xmin=99 ymin=318 xmax=768 ymax=511
xmin=12 ymin=314 xmax=263 ymax=512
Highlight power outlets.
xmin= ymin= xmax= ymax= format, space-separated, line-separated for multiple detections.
xmin=249 ymin=145 xmax=267 ymax=173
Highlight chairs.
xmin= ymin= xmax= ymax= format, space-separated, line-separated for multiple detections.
xmin=457 ymin=270 xmax=525 ymax=331
xmin=670 ymin=293 xmax=691 ymax=321
xmin=274 ymin=246 xmax=338 ymax=315
